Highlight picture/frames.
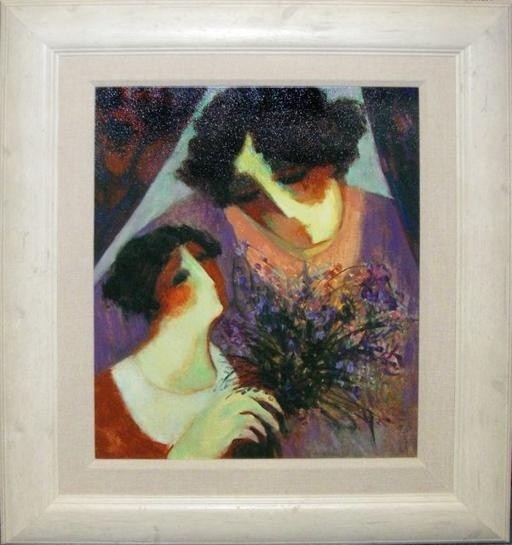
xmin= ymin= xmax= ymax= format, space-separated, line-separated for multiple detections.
xmin=1 ymin=0 xmax=512 ymax=544
